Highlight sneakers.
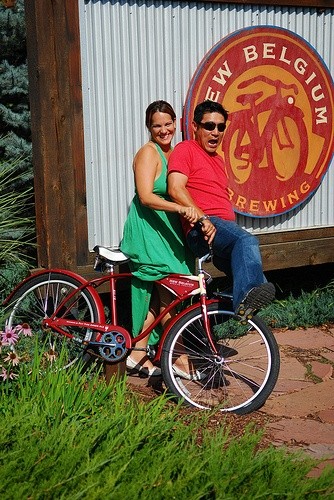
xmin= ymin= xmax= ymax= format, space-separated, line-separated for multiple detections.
xmin=234 ymin=282 xmax=275 ymax=327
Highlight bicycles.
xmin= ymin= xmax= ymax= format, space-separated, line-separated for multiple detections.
xmin=2 ymin=219 xmax=280 ymax=415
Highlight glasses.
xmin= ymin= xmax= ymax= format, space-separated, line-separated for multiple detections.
xmin=195 ymin=122 xmax=227 ymax=132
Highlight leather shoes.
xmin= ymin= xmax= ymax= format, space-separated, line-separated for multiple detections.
xmin=171 ymin=365 xmax=207 ymax=381
xmin=126 ymin=356 xmax=161 ymax=376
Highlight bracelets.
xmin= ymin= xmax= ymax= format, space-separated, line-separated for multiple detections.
xmin=198 ymin=215 xmax=209 ymax=225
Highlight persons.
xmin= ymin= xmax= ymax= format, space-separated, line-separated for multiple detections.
xmin=119 ymin=100 xmax=207 ymax=380
xmin=167 ymin=100 xmax=275 ymax=390
